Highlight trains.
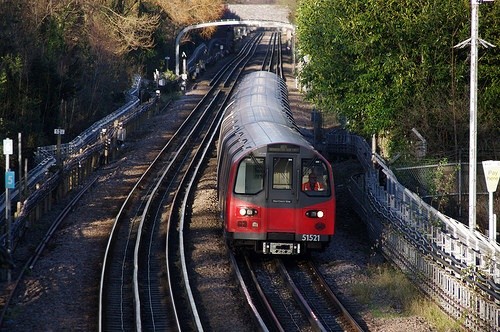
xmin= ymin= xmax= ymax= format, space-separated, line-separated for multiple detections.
xmin=217 ymin=71 xmax=335 ymax=256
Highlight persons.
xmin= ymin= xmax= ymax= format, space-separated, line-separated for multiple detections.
xmin=302 ymin=173 xmax=327 ymax=191
